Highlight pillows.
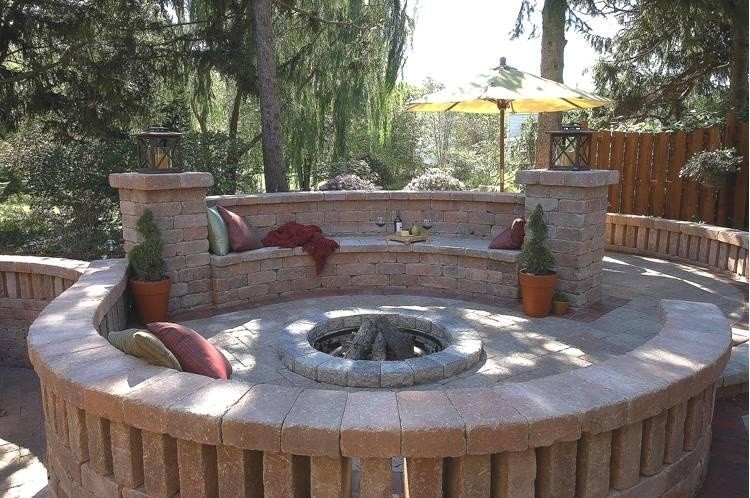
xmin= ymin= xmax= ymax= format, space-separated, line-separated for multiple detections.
xmin=218 ymin=207 xmax=263 ymax=251
xmin=489 ymin=217 xmax=524 ymax=249
xmin=108 ymin=329 xmax=182 ymax=371
xmin=146 ymin=322 xmax=232 ymax=379
xmin=207 ymin=205 xmax=229 ymax=256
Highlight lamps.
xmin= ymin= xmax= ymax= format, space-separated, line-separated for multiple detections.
xmin=135 ymin=127 xmax=183 ymax=174
xmin=546 ymin=123 xmax=592 ymax=171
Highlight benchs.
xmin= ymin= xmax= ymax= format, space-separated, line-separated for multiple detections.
xmin=206 ymin=190 xmax=524 ymax=309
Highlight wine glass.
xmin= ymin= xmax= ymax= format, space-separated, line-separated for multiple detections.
xmin=422 ymin=219 xmax=434 ymax=245
xmin=375 ymin=216 xmax=386 ymax=241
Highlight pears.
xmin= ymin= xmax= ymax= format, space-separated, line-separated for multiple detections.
xmin=411 ymin=225 xmax=420 ymax=235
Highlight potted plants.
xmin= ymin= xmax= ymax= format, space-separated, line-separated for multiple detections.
xmin=553 ymin=292 xmax=569 ymax=315
xmin=678 ymin=146 xmax=746 ymax=190
xmin=519 ymin=204 xmax=557 ymax=318
xmin=128 ymin=207 xmax=172 ymax=323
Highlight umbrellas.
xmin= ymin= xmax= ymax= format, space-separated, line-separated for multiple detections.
xmin=404 ymin=56 xmax=613 ymax=195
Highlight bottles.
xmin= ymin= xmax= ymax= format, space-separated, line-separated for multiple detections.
xmin=394 ymin=208 xmax=403 ymax=235
